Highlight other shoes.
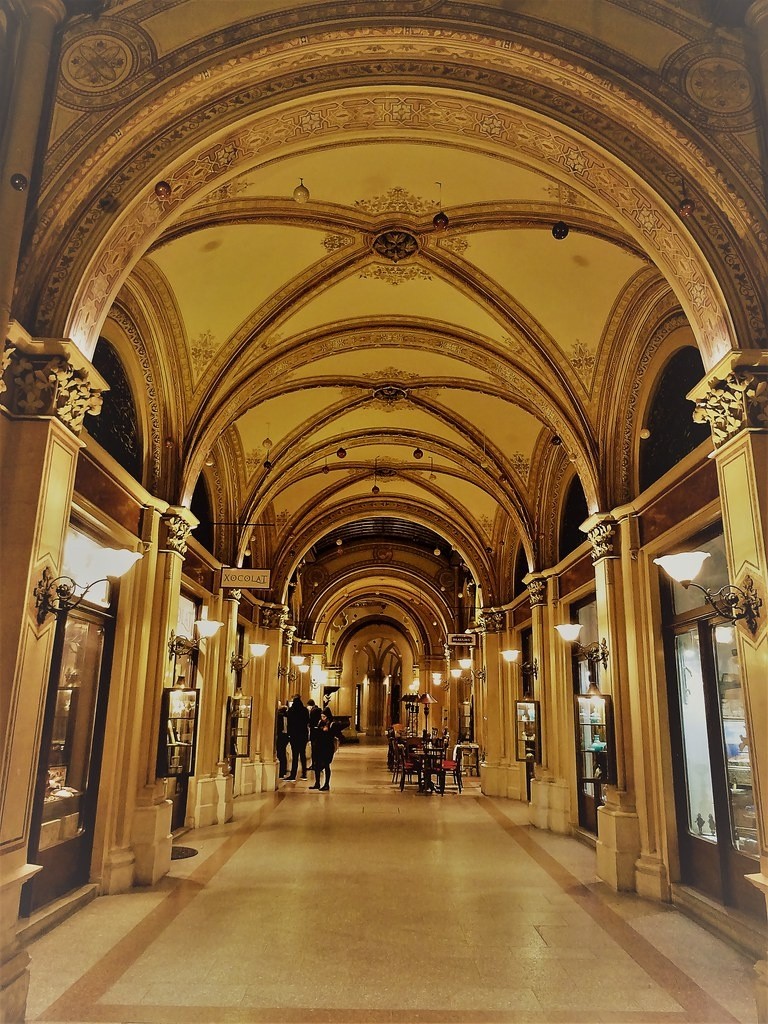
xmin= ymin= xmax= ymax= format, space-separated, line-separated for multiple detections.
xmin=300 ymin=776 xmax=307 ymax=778
xmin=309 ymin=783 xmax=320 ymax=789
xmin=278 ymin=777 xmax=284 ymax=779
xmin=305 ymin=766 xmax=313 ymax=770
xmin=284 ymin=774 xmax=286 ymax=776
xmin=282 ymin=776 xmax=296 ymax=782
xmin=318 ymin=784 xmax=329 ymax=790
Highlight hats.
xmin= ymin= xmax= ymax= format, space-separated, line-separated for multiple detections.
xmin=306 ymin=699 xmax=314 ymax=706
xmin=292 ymin=694 xmax=301 ymax=699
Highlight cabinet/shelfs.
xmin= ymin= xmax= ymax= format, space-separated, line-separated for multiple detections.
xmin=48 ymin=686 xmax=79 ymax=786
xmin=514 ymin=700 xmax=541 ymax=763
xmin=224 ymin=695 xmax=253 ymax=758
xmin=155 ymin=688 xmax=201 ymax=777
xmin=574 ymin=694 xmax=616 ymax=785
xmin=458 ymin=702 xmax=474 ymax=742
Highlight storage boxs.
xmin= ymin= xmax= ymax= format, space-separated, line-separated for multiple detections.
xmin=49 ymin=765 xmax=78 ymax=794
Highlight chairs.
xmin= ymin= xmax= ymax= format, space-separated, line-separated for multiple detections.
xmin=424 ymin=737 xmax=450 ymax=775
xmin=398 ymin=744 xmax=423 ymax=793
xmin=387 ymin=738 xmax=413 ymax=783
xmin=437 ymin=746 xmax=462 ymax=793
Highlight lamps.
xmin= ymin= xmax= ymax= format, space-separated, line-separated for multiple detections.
xmin=554 ymin=623 xmax=610 ymax=670
xmin=310 ymin=664 xmax=328 ymax=690
xmin=498 ymin=650 xmax=538 ymax=680
xmin=458 ymin=659 xmax=486 ymax=682
xmin=399 ymin=678 xmax=439 ymax=738
xmin=168 ymin=620 xmax=224 ymax=660
xmin=450 ymin=670 xmax=473 ymax=687
xmin=653 ymin=551 xmax=762 ymax=636
xmin=288 ymin=665 xmax=310 ymax=683
xmin=431 ymin=673 xmax=449 ymax=692
xmin=33 ymin=547 xmax=144 ymax=626
xmin=230 ymin=644 xmax=270 ymax=673
xmin=278 ymin=656 xmax=307 ymax=679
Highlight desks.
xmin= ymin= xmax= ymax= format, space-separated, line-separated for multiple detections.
xmin=401 ymin=736 xmax=430 ymax=746
xmin=456 ymin=747 xmax=480 ymax=777
xmin=409 ymin=755 xmax=445 ymax=794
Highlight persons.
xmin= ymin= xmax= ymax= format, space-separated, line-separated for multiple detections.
xmin=276 ymin=694 xmax=337 ymax=791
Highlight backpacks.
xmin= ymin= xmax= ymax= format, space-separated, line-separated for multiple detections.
xmin=317 ymin=719 xmax=339 ymax=753
